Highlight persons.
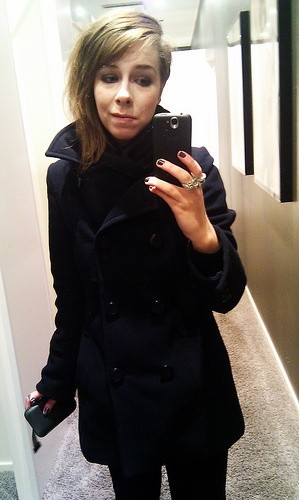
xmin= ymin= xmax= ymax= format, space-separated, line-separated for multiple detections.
xmin=25 ymin=14 xmax=247 ymax=500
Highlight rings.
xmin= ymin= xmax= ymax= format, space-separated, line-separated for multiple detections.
xmin=27 ymin=394 xmax=37 ymax=406
xmin=181 ymin=180 xmax=199 ymax=190
xmin=190 ymin=172 xmax=207 ymax=185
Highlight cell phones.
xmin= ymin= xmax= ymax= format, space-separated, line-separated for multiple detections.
xmin=152 ymin=112 xmax=192 ymax=187
